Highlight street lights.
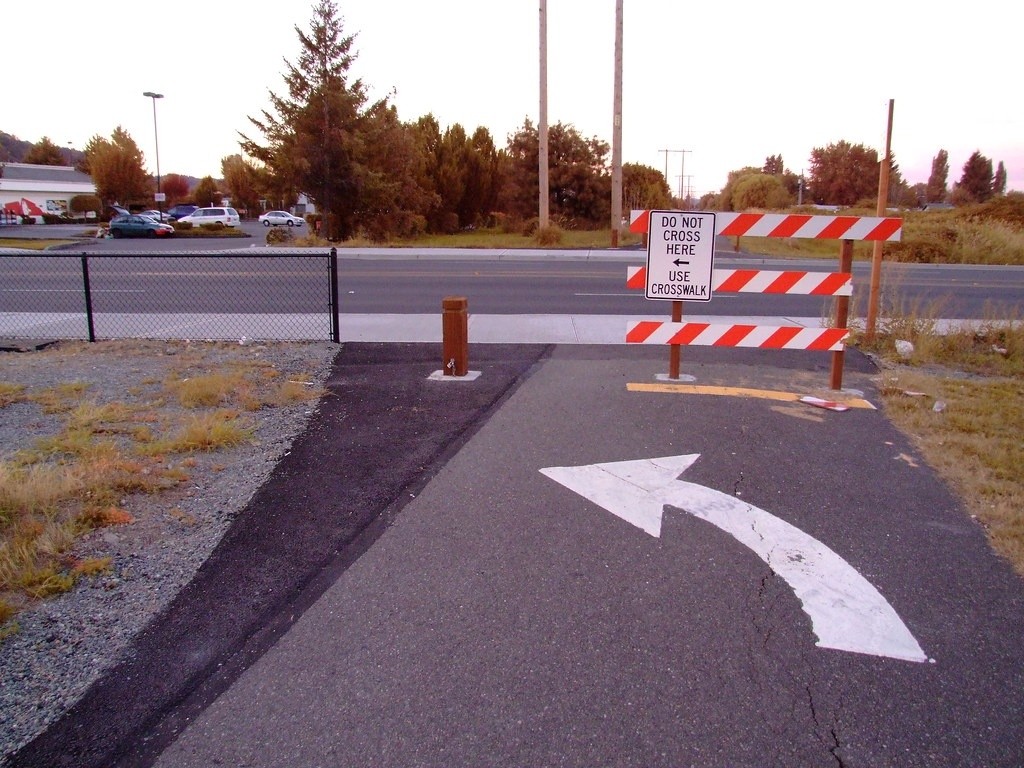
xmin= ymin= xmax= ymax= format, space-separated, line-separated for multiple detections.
xmin=144 ymin=91 xmax=165 ymax=222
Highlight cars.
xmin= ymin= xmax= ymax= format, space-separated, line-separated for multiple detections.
xmin=138 ymin=210 xmax=169 ymax=223
xmin=258 ymin=210 xmax=305 ymax=227
xmin=18 ymin=215 xmax=36 ymax=225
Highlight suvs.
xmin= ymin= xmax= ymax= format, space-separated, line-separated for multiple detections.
xmin=104 ymin=205 xmax=175 ymax=238
xmin=167 ymin=205 xmax=200 ymax=219
xmin=177 ymin=206 xmax=241 ymax=228
